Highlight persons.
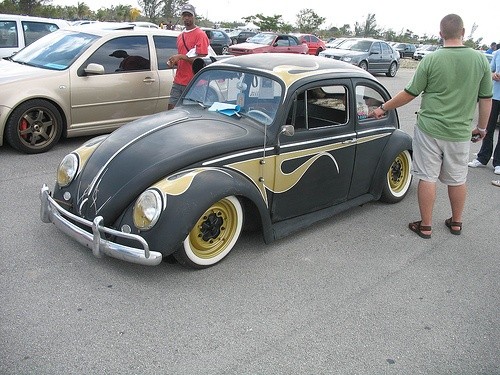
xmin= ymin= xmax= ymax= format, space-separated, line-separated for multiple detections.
xmin=486 ymin=44 xmax=500 ymax=53
xmin=165 ymin=8 xmax=210 ymax=110
xmin=160 ymin=20 xmax=164 ymax=29
xmin=110 ymin=50 xmax=151 ymax=72
xmin=367 ymin=13 xmax=495 ymax=239
xmin=468 ymin=46 xmax=500 ymax=175
xmin=166 ymin=21 xmax=174 ymax=30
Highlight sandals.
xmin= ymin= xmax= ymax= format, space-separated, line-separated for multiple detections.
xmin=445 ymin=216 xmax=462 ymax=235
xmin=409 ymin=220 xmax=432 ymax=239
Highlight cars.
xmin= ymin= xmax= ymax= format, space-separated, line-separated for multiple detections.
xmin=0 ymin=12 xmax=487 ymax=56
xmin=38 ymin=51 xmax=414 ymax=270
xmin=318 ymin=37 xmax=400 ymax=77
xmin=0 ymin=24 xmax=222 ymax=155
xmin=225 ymin=31 xmax=309 ymax=56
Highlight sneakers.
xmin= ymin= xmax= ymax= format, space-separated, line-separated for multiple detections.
xmin=468 ymin=159 xmax=487 ymax=168
xmin=493 ymin=166 xmax=500 ymax=175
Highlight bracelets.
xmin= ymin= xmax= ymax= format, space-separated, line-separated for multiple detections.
xmin=179 ymin=54 xmax=182 ymax=59
xmin=476 ymin=125 xmax=487 ymax=132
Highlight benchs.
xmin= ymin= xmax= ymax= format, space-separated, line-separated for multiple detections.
xmin=292 ymin=101 xmax=347 ymax=129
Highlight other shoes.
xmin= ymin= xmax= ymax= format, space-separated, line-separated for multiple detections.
xmin=491 ymin=180 xmax=500 ymax=187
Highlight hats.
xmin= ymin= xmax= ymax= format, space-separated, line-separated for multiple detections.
xmin=109 ymin=50 xmax=127 ymax=57
xmin=181 ymin=5 xmax=195 ymax=17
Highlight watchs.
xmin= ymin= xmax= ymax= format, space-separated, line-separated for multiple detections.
xmin=379 ymin=103 xmax=386 ymax=113
